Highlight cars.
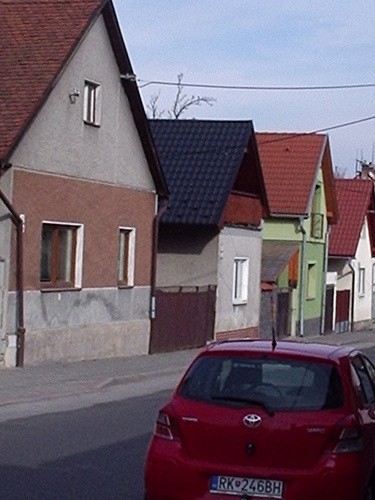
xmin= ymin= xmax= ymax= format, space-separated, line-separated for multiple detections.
xmin=141 ymin=337 xmax=375 ymax=500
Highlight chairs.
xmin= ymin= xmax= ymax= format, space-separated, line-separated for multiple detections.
xmin=222 ymin=366 xmax=268 ymax=403
